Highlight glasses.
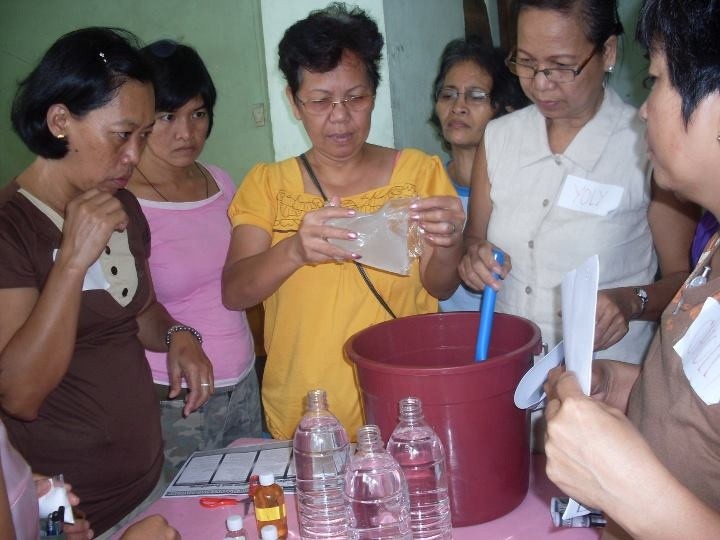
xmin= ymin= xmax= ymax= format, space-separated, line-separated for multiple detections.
xmin=435 ymin=86 xmax=493 ymax=106
xmin=504 ymin=43 xmax=599 ymax=84
xmin=292 ymin=91 xmax=374 ymax=117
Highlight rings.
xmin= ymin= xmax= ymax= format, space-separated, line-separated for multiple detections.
xmin=201 ymin=384 xmax=210 ymax=386
xmin=453 ymin=225 xmax=455 ymax=232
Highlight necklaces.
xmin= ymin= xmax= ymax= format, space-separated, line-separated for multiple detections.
xmin=135 ymin=161 xmax=209 ymax=201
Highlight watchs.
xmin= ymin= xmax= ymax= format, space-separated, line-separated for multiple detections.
xmin=632 ymin=286 xmax=648 ymax=320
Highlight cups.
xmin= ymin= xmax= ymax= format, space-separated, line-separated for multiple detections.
xmin=39 ymin=474 xmax=74 ymax=523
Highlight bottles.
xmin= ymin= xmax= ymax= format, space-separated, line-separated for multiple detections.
xmin=224 ymin=515 xmax=249 ymax=540
xmin=343 ymin=424 xmax=413 ymax=540
xmin=261 ymin=526 xmax=279 ymax=540
xmin=292 ymin=390 xmax=352 ymax=540
xmin=387 ymin=397 xmax=454 ymax=540
xmin=253 ymin=471 xmax=288 ymax=540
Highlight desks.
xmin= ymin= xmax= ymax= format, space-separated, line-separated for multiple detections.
xmin=114 ymin=437 xmax=604 ymax=540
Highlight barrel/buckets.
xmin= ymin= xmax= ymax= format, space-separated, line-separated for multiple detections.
xmin=344 ymin=311 xmax=542 ymax=528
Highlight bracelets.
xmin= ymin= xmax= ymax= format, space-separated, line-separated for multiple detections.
xmin=167 ymin=325 xmax=202 ymax=345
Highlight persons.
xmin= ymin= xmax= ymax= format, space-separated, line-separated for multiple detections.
xmin=546 ymin=0 xmax=719 ymax=540
xmin=456 ymin=0 xmax=703 ymax=366
xmin=222 ymin=0 xmax=467 ymax=438
xmin=124 ymin=40 xmax=263 ymax=483
xmin=424 ymin=38 xmax=528 ymax=310
xmin=0 ymin=25 xmax=215 ymax=540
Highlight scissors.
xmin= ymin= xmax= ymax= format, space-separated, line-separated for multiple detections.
xmin=199 ymin=475 xmax=259 ymax=519
